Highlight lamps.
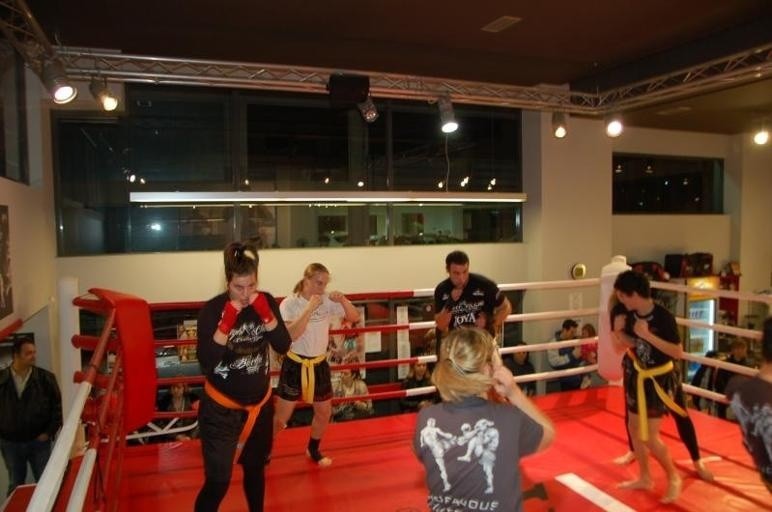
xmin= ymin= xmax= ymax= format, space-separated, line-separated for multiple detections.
xmin=39 ymin=56 xmax=77 ymax=104
xmin=117 ymin=168 xmax=529 ymax=211
xmin=437 ymin=96 xmax=459 ymax=133
xmin=89 ymin=76 xmax=118 ymax=111
xmin=550 ymin=112 xmax=566 ymax=139
xmin=358 ymin=97 xmax=376 ymax=124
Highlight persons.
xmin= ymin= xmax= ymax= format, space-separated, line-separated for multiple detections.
xmin=273 ymin=262 xmax=361 ymax=468
xmin=456 ymin=419 xmax=500 ymax=494
xmin=419 ymin=417 xmax=481 ymax=492
xmin=691 ymin=316 xmax=772 ymax=493
xmin=609 ymin=283 xmax=716 ymax=482
xmin=545 ymin=318 xmax=597 ymax=391
xmin=578 ymin=323 xmax=599 ymax=390
xmin=612 ymin=269 xmax=685 ymax=504
xmin=163 ymin=374 xmax=196 ymax=441
xmin=332 ymin=368 xmax=374 ymax=418
xmin=193 ymin=241 xmax=292 ymax=512
xmin=412 ymin=327 xmax=554 ymax=511
xmin=399 ymin=251 xmax=538 ymax=414
xmin=0 ymin=338 xmax=64 ymax=498
